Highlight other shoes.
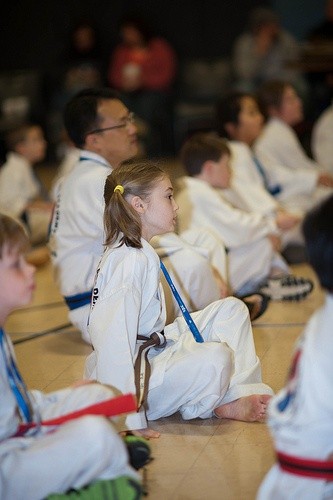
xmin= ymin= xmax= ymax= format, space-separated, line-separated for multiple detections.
xmin=258 ymin=274 xmax=314 ymax=302
xmin=43 ymin=475 xmax=144 ymax=500
xmin=122 ymin=436 xmax=152 ymax=469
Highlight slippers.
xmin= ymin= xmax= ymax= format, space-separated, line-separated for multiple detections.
xmin=239 ymin=292 xmax=269 ymax=322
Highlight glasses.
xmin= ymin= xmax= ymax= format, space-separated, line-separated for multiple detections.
xmin=86 ymin=111 xmax=134 ymax=137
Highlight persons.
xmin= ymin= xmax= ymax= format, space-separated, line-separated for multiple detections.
xmin=83 ymin=157 xmax=275 ymax=440
xmin=0 ymin=0 xmax=333 ymax=500
